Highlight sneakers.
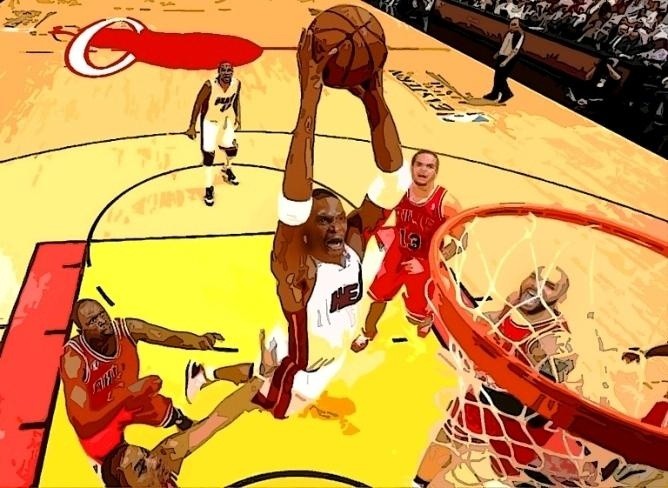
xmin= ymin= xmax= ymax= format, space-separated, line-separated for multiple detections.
xmin=184 ymin=359 xmax=204 ymax=404
xmin=498 ymin=92 xmax=513 ymax=104
xmin=203 ymin=185 xmax=217 ymax=207
xmin=417 ymin=321 xmax=432 ymax=338
xmin=350 ymin=332 xmax=370 ymax=354
xmin=482 ymin=93 xmax=499 ymax=101
xmin=177 ymin=419 xmax=199 ymax=432
xmin=220 ymin=168 xmax=240 ymax=186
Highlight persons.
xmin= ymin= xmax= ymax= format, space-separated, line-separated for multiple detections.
xmin=58 ymin=294 xmax=225 ymax=464
xmin=411 ymin=263 xmax=584 ymax=487
xmin=98 ymin=326 xmax=278 ymax=486
xmin=363 ymin=0 xmax=666 ymax=159
xmin=600 ymin=339 xmax=668 ymax=487
xmin=348 ymin=148 xmax=467 ymax=356
xmin=185 ymin=57 xmax=242 ymax=205
xmin=180 ymin=25 xmax=410 ymax=418
